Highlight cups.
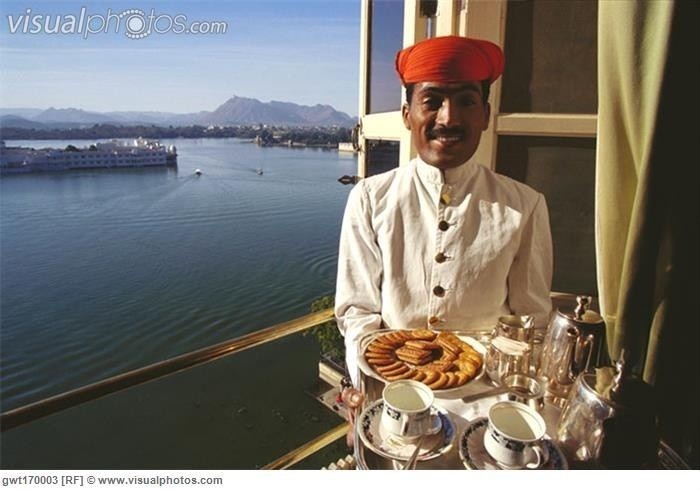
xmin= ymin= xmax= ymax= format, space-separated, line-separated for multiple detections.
xmin=378 ymin=315 xmax=547 ymax=470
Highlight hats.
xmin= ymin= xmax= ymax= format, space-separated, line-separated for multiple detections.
xmin=394 ymin=35 xmax=505 ymax=87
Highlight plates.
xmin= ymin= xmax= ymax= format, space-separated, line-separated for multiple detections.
xmin=358 ymin=398 xmax=457 ymax=462
xmin=356 ymin=330 xmax=487 ymax=392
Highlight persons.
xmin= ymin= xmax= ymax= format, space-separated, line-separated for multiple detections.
xmin=335 ymin=35 xmax=555 ymax=449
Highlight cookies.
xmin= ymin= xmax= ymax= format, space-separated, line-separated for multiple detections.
xmin=364 ymin=329 xmax=484 ymax=389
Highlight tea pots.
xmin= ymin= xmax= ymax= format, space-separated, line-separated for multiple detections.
xmin=535 ymin=296 xmax=665 ymax=470
